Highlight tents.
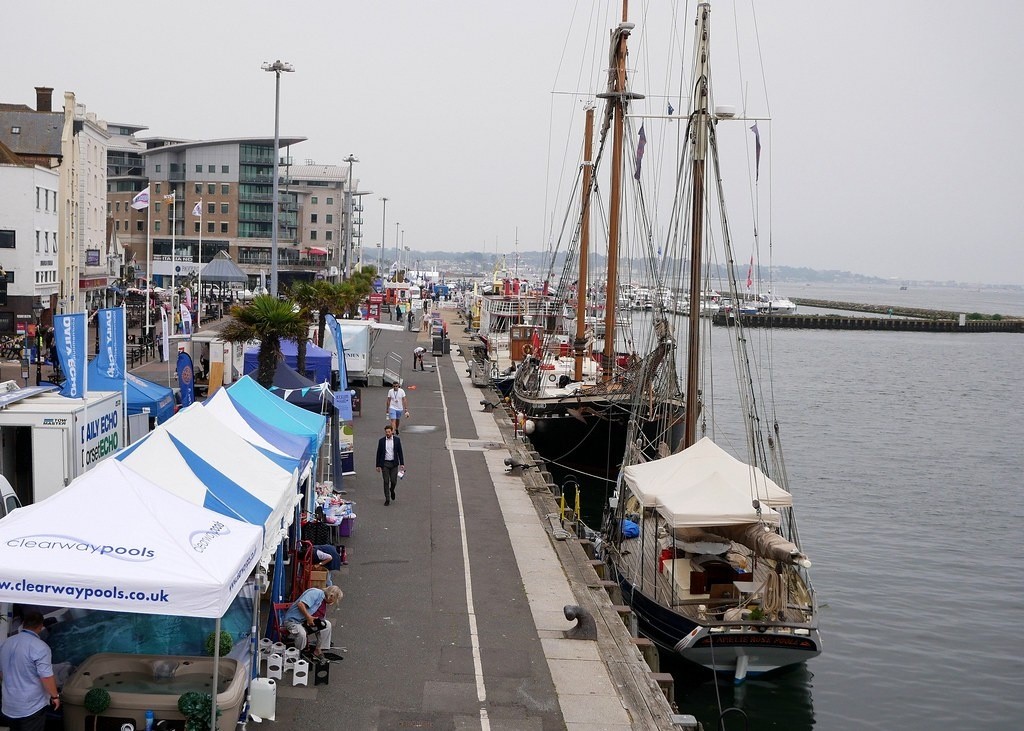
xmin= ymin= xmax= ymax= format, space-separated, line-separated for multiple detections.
xmin=57 ymin=353 xmax=175 ymax=426
xmin=0 ymin=456 xmax=267 ymax=731
xmin=76 ymin=427 xmax=285 ymax=623
xmin=623 ymin=435 xmax=794 ymax=611
xmin=243 ymin=340 xmax=332 ymax=391
xmin=178 ymin=373 xmax=326 ymax=522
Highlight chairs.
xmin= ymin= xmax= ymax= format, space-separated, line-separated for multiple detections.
xmin=272 ymin=601 xmax=310 ymax=652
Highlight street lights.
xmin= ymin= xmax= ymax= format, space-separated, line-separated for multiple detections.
xmin=378 ymin=194 xmax=390 ymax=273
xmin=395 ymin=222 xmax=411 ymax=268
xmin=261 ymin=58 xmax=296 ymax=294
xmin=341 ymin=153 xmax=360 ymax=280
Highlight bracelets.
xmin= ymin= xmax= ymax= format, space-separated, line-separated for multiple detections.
xmin=51 ymin=696 xmax=59 ymax=699
xmin=319 ymin=563 xmax=323 ymax=566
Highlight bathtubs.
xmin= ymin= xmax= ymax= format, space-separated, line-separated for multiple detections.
xmin=60 ymin=652 xmax=247 ymax=731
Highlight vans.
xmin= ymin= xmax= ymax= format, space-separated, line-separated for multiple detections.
xmin=194 ymin=283 xmax=254 ymax=302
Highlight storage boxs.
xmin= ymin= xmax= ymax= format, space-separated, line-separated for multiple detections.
xmin=305 ymin=565 xmax=329 ymax=591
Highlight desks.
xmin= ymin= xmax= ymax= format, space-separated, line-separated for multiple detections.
xmin=325 ymin=516 xmax=344 ymax=545
xmin=732 ymin=581 xmax=764 ymax=608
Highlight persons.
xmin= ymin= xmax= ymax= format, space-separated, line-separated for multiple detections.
xmin=386 ymin=381 xmax=411 ymax=435
xmin=422 ymin=283 xmax=453 ymax=332
xmin=41 ymin=320 xmax=57 ymax=381
xmin=375 ymin=424 xmax=405 ymax=506
xmin=298 ymin=543 xmax=341 ymax=574
xmin=387 ymin=303 xmax=404 ymax=322
xmin=413 ymin=346 xmax=427 ymax=372
xmin=408 ymin=309 xmax=415 ymax=332
xmin=467 ymin=310 xmax=473 ymax=329
xmin=284 ymin=585 xmax=343 ymax=661
xmin=0 ymin=611 xmax=60 ymax=731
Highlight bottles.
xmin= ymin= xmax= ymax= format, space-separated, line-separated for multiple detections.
xmin=386 ymin=412 xmax=389 ymax=420
xmin=145 ymin=711 xmax=153 ymax=731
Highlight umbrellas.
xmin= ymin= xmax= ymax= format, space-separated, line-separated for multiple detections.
xmin=300 ymin=244 xmax=327 ymax=260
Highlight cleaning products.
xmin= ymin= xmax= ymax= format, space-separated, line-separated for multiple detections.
xmin=145 ymin=710 xmax=154 ymax=731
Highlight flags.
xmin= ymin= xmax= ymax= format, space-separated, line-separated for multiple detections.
xmin=192 ymin=202 xmax=203 ymax=216
xmin=163 ymin=192 xmax=174 ymax=206
xmin=131 ymin=186 xmax=149 ymax=209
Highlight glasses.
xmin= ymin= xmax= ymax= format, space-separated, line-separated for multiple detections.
xmin=393 ymin=386 xmax=398 ymax=387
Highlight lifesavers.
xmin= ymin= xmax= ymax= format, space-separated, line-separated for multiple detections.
xmin=522 ymin=344 xmax=534 ymax=357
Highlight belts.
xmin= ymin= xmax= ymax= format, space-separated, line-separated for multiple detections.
xmin=385 ymin=460 xmax=394 ymax=462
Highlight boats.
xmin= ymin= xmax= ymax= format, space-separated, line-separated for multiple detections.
xmin=442 ymin=225 xmax=800 ymax=394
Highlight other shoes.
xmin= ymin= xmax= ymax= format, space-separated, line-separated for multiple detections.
xmin=314 ymin=653 xmax=325 ymax=659
xmin=384 ymin=499 xmax=390 ymax=506
xmin=389 ymin=488 xmax=395 ymax=500
xmin=396 ymin=430 xmax=399 ymax=435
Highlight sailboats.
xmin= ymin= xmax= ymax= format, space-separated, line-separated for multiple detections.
xmin=504 ymin=0 xmax=702 ymax=460
xmin=597 ymin=0 xmax=826 ymax=680
